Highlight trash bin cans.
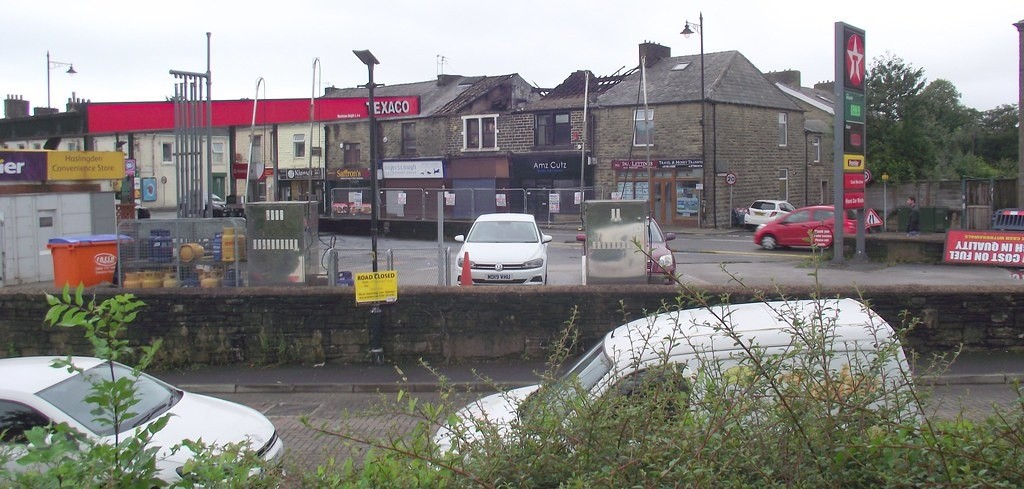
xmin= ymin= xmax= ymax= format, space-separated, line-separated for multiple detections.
xmin=935 ymin=206 xmax=948 ymax=233
xmin=45 ymin=234 xmax=134 ymax=289
xmin=918 ymin=207 xmax=934 ymax=230
xmin=138 ymin=207 xmax=151 ymax=219
xmin=226 ymin=204 xmax=244 ymax=217
xmin=897 ymin=206 xmax=911 ymax=231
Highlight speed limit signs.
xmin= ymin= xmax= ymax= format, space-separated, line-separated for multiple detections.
xmin=726 ymin=172 xmax=736 ymax=185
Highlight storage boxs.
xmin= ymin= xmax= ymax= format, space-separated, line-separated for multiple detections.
xmin=46 ymin=234 xmax=134 ymax=289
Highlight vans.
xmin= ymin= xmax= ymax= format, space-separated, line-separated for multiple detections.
xmin=181 ymin=194 xmax=226 ymax=218
xmin=429 ymin=300 xmax=921 ymax=480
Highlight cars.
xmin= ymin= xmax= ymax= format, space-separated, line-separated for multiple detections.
xmin=754 ymin=206 xmax=868 ymax=250
xmin=744 ymin=200 xmax=796 ymax=230
xmin=455 ymin=213 xmax=553 ymax=286
xmin=577 ymin=222 xmax=677 ymax=285
xmin=0 ymin=356 xmax=286 ymax=489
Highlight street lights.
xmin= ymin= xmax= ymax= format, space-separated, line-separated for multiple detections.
xmin=680 ymin=13 xmax=708 ymax=226
xmin=353 ymin=49 xmax=385 ymax=363
xmin=47 ymin=52 xmax=76 ymax=110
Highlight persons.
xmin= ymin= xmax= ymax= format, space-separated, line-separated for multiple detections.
xmin=906 ymin=197 xmax=920 ymax=233
